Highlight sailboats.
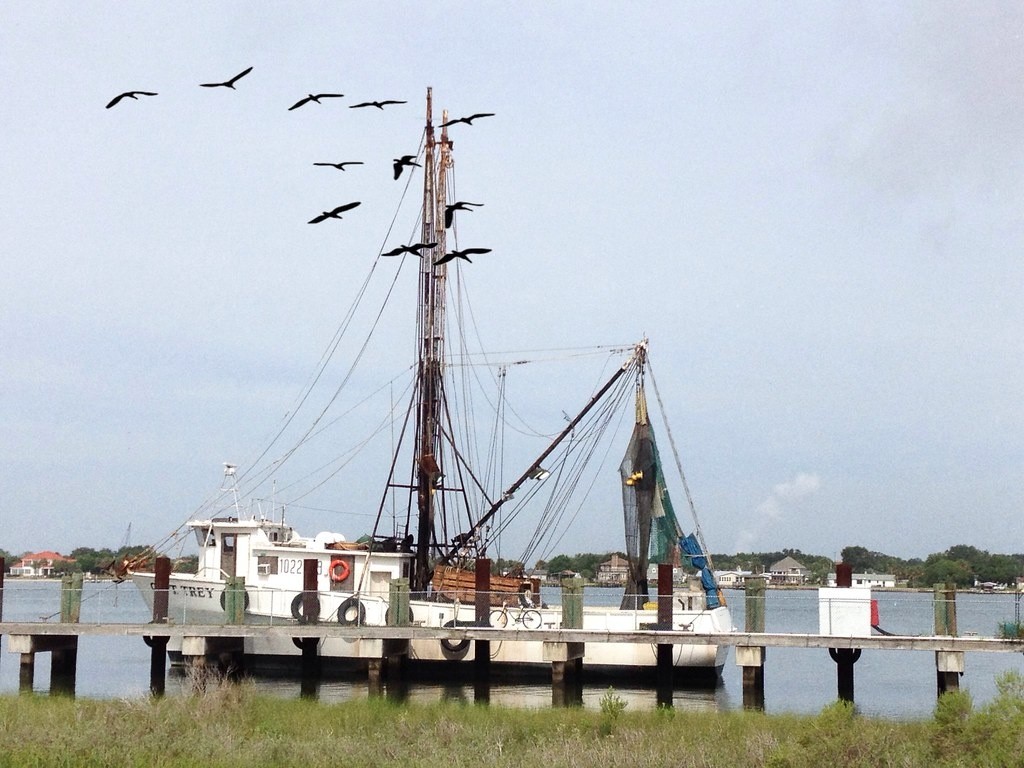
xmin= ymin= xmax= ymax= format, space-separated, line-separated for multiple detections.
xmin=122 ymin=83 xmax=736 ymax=691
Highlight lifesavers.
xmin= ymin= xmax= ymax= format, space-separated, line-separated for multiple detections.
xmin=291 ymin=637 xmax=321 ymax=649
xmin=328 ymin=560 xmax=351 ymax=582
xmin=384 ymin=604 xmax=414 ymax=627
xmin=440 ymin=620 xmax=471 ymax=652
xmin=220 ymin=585 xmax=249 ymax=612
xmin=290 ymin=593 xmax=321 ymax=623
xmin=337 ymin=597 xmax=366 ymax=627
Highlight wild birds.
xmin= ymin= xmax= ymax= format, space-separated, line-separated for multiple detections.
xmin=288 ymin=93 xmax=344 ymax=112
xmin=438 ymin=113 xmax=496 ymax=129
xmin=349 ymin=100 xmax=407 ymax=110
xmin=381 ymin=242 xmax=439 ymax=258
xmin=199 ymin=66 xmax=254 ymax=90
xmin=313 ymin=160 xmax=364 ymax=171
xmin=432 ymin=247 xmax=492 ymax=266
xmin=443 ymin=201 xmax=484 ymax=228
xmin=307 ymin=201 xmax=361 ymax=224
xmin=106 ymin=91 xmax=159 ymax=110
xmin=392 ymin=155 xmax=423 ymax=180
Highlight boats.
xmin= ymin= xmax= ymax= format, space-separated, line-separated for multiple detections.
xmin=439 ymin=365 xmax=520 ymax=605
xmin=430 ymin=364 xmax=520 ymax=605
